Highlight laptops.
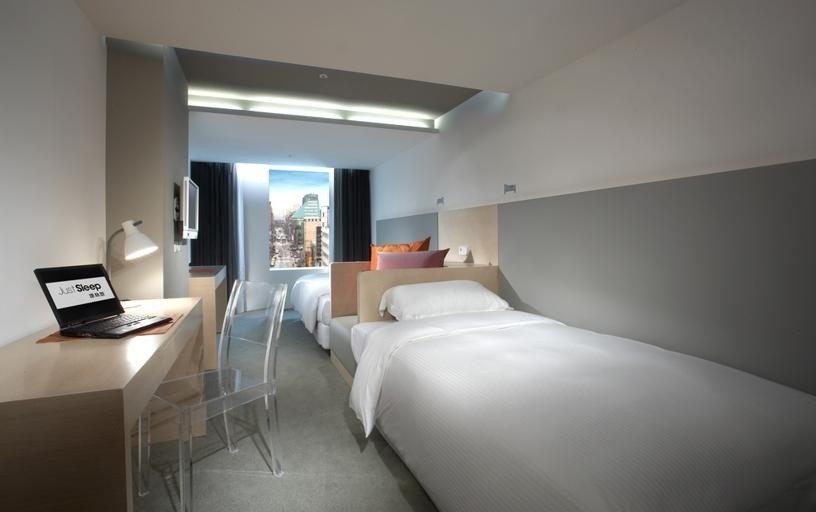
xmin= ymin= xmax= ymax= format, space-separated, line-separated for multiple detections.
xmin=33 ymin=264 xmax=173 ymax=339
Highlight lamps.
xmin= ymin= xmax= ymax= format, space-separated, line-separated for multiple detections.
xmin=105 ymin=218 xmax=159 ymax=309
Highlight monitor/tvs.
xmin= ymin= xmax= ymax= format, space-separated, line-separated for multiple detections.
xmin=180 ymin=177 xmax=200 ymax=240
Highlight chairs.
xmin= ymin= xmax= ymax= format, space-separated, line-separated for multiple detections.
xmin=136 ymin=278 xmax=289 ymax=512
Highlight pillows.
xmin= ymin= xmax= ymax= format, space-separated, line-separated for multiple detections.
xmin=369 ymin=236 xmax=450 ymax=271
